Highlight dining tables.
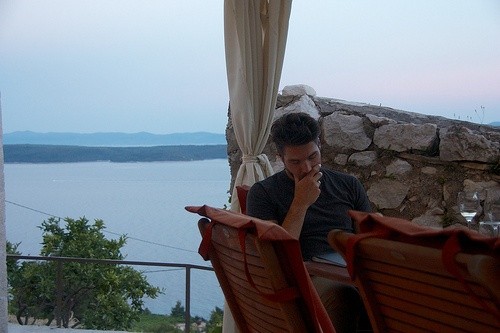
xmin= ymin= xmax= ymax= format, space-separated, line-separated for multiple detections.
xmin=304 ymin=260 xmax=354 ymax=284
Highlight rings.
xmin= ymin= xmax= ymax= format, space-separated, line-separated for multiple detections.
xmin=317 ymin=180 xmax=322 ymax=185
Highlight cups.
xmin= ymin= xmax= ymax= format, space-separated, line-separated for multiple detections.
xmin=479 ymin=222 xmax=500 ymax=236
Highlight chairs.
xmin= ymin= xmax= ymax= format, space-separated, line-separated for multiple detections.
xmin=185 ymin=205 xmax=337 ymax=333
xmin=328 ymin=210 xmax=500 ymax=333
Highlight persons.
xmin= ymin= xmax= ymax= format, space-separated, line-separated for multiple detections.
xmin=246 ymin=112 xmax=385 ymax=332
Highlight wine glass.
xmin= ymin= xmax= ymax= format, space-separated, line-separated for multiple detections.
xmin=458 ymin=192 xmax=479 ymax=230
